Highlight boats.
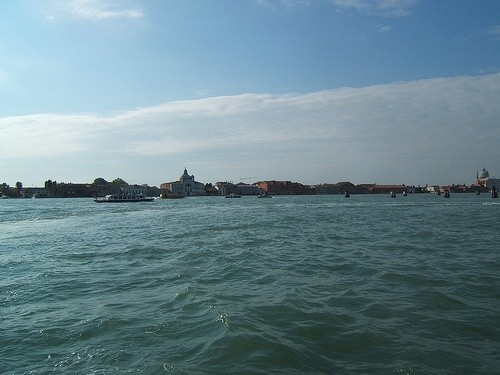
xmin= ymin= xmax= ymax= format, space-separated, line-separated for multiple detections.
xmin=94 ymin=194 xmax=154 ymax=204
xmin=257 ymin=194 xmax=271 ymax=198
xmin=225 ymin=194 xmax=241 ymax=198
